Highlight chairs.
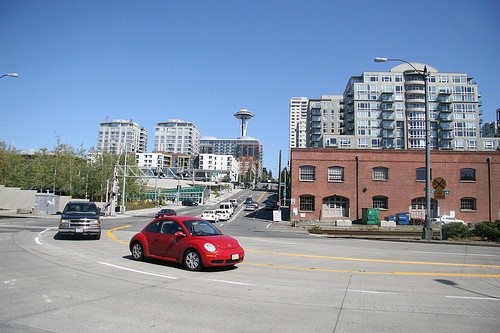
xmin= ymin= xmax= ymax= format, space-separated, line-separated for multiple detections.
xmin=69 ymin=208 xmax=77 ymax=211
xmin=162 ymin=223 xmax=176 ymax=234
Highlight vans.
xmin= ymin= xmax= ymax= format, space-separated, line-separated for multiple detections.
xmin=219 ymin=202 xmax=233 ymax=215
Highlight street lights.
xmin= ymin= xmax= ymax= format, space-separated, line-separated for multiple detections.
xmin=374 ymin=57 xmax=433 ymax=240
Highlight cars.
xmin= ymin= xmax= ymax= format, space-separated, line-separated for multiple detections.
xmin=129 ymin=216 xmax=245 ymax=271
xmin=56 ymin=201 xmax=105 ymax=240
xmin=431 ymin=215 xmax=465 ymax=225
xmin=155 ymin=209 xmax=177 ymax=222
xmin=202 ymin=210 xmax=231 ymax=223
xmin=243 ymin=197 xmax=259 ymax=210
xmin=182 ymin=199 xmax=198 ymax=206
xmin=228 ymin=199 xmax=238 ymax=211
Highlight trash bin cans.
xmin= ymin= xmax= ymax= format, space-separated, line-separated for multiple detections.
xmin=385 ymin=215 xmax=396 ymax=221
xmin=396 ymin=212 xmax=409 ymax=224
xmin=362 ymin=208 xmax=380 ymax=225
